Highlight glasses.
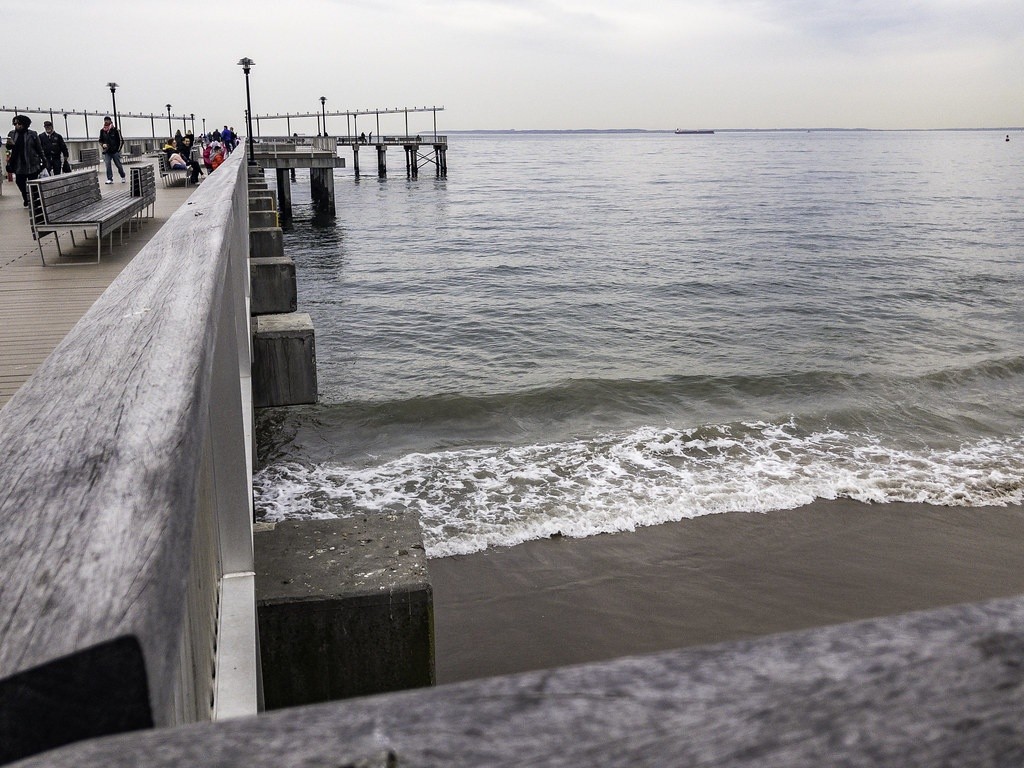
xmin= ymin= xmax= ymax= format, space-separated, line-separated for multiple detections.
xmin=13 ymin=122 xmax=22 ymax=126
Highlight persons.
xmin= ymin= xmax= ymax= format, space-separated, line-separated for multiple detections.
xmin=162 ymin=124 xmax=240 ymax=186
xmin=98 ymin=116 xmax=126 ymax=184
xmin=5 ymin=115 xmax=47 ymax=207
xmin=38 ymin=121 xmax=70 ymax=177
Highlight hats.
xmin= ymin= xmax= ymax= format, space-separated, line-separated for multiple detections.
xmin=43 ymin=121 xmax=52 ymax=127
xmin=213 ymin=146 xmax=221 ymax=150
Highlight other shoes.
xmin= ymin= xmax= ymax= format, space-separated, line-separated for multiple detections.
xmin=23 ymin=201 xmax=28 ymax=207
xmin=195 ymin=181 xmax=202 ymax=185
xmin=105 ymin=179 xmax=114 ymax=184
xmin=189 ymin=167 xmax=193 ymax=171
xmin=187 ymin=165 xmax=191 ymax=169
xmin=121 ymin=177 xmax=126 ymax=183
xmin=201 ymin=174 xmax=207 ymax=178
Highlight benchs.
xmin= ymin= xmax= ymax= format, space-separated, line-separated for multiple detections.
xmin=158 ymin=146 xmax=204 ymax=188
xmin=25 ymin=163 xmax=155 ymax=267
xmin=71 ymin=143 xmax=159 ymax=172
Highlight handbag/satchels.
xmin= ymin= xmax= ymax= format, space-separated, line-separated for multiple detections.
xmin=62 ymin=159 xmax=72 ymax=173
xmin=6 ymin=165 xmax=14 ymax=173
